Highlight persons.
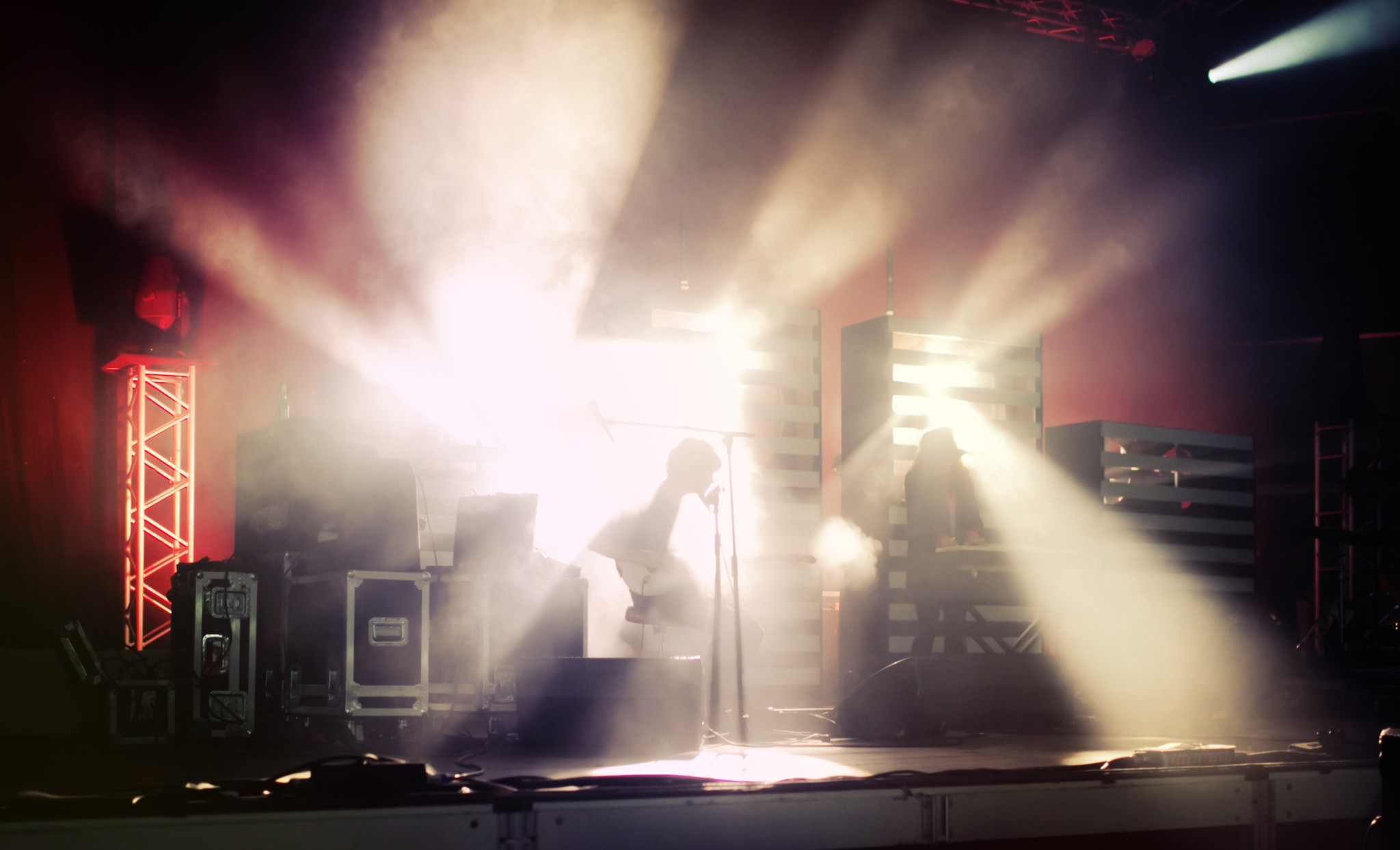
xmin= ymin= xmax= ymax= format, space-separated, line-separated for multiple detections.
xmin=905 ymin=428 xmax=987 ymax=658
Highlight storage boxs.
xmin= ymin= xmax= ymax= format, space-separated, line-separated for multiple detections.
xmin=426 ymin=565 xmax=589 ymax=712
xmin=56 ymin=618 xmax=175 ymax=747
xmin=170 ymin=569 xmax=258 ymax=738
xmin=254 ymin=551 xmax=431 ymax=718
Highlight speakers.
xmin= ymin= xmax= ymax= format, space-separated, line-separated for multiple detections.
xmin=815 ymin=650 xmax=1084 ymax=744
xmin=518 ymin=652 xmax=708 ymax=753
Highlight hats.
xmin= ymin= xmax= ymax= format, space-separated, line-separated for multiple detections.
xmin=913 ymin=428 xmax=965 ymax=469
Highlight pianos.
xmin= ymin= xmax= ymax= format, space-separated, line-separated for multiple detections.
xmin=933 ymin=545 xmax=1056 ymax=655
